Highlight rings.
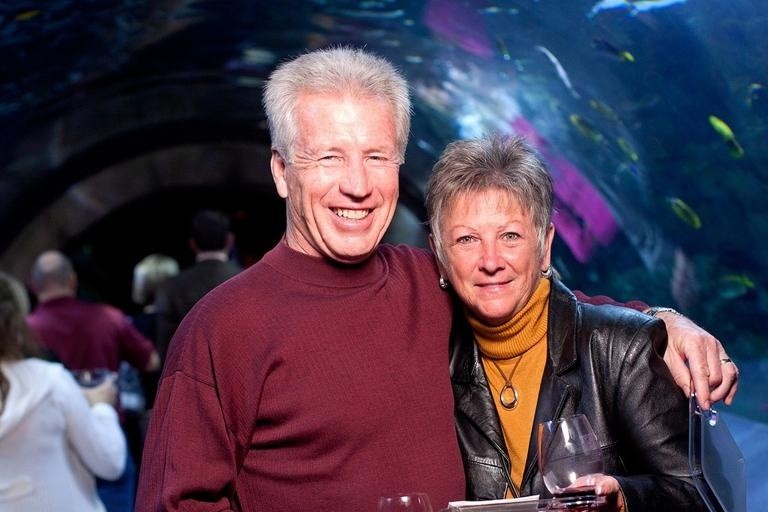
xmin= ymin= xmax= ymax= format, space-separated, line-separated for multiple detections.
xmin=720 ymin=357 xmax=731 ymax=365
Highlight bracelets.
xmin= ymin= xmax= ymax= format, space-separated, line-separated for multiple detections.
xmin=643 ymin=305 xmax=684 ymax=317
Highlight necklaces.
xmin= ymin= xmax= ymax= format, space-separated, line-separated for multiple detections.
xmin=489 ymin=352 xmax=522 ymax=408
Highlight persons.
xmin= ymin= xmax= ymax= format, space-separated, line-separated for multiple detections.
xmin=152 ymin=211 xmax=249 ymax=361
xmin=133 ymin=43 xmax=739 ymax=511
xmin=118 ymin=252 xmax=187 ymax=407
xmin=20 ymin=251 xmax=165 ymax=511
xmin=0 ymin=270 xmax=127 ymax=510
xmin=420 ymin=129 xmax=745 ymax=512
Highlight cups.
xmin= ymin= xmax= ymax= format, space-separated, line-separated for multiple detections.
xmin=376 ymin=492 xmax=433 ymax=512
xmin=75 ymin=367 xmax=109 ymax=388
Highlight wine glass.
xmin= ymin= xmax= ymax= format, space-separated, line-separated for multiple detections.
xmin=536 ymin=413 xmax=608 ymax=512
